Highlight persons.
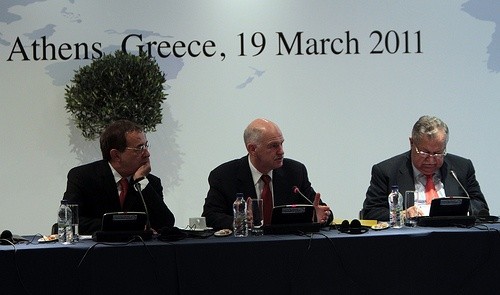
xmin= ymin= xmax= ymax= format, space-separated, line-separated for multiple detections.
xmin=61 ymin=120 xmax=175 ymax=236
xmin=201 ymin=118 xmax=333 ymax=231
xmin=363 ymin=115 xmax=489 ymax=222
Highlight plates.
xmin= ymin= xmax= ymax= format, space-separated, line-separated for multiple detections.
xmin=371 ymin=224 xmax=389 ymax=229
xmin=38 ymin=234 xmax=59 ymax=242
xmin=214 ymin=230 xmax=232 ymax=236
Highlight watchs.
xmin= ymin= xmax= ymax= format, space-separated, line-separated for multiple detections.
xmin=134 ymin=176 xmax=146 ymax=184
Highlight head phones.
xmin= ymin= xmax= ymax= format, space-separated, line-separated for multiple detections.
xmin=337 ymin=220 xmax=370 ymax=235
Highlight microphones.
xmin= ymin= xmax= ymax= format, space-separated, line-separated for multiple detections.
xmin=409 ymin=169 xmax=475 ymax=228
xmin=264 ymin=185 xmax=322 ymax=234
xmin=90 ymin=181 xmax=152 ymax=241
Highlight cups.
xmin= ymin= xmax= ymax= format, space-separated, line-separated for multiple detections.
xmin=66 ymin=204 xmax=79 ymax=239
xmin=250 ymin=199 xmax=264 ymax=236
xmin=189 ymin=217 xmax=206 ymax=230
xmin=405 ymin=191 xmax=418 ymax=228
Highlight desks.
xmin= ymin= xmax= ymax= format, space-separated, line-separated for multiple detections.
xmin=0 ymin=217 xmax=500 ymax=295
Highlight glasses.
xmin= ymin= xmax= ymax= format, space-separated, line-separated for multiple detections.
xmin=413 ymin=142 xmax=447 ymax=160
xmin=124 ymin=143 xmax=150 ymax=153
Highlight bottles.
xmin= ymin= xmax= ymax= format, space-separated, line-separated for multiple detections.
xmin=57 ymin=200 xmax=73 ymax=245
xmin=233 ymin=193 xmax=248 ymax=238
xmin=388 ymin=186 xmax=404 ymax=229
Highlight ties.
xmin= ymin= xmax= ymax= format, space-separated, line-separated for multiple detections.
xmin=259 ymin=173 xmax=273 ymax=225
xmin=425 ymin=173 xmax=438 ymax=205
xmin=118 ymin=178 xmax=129 ymax=205
